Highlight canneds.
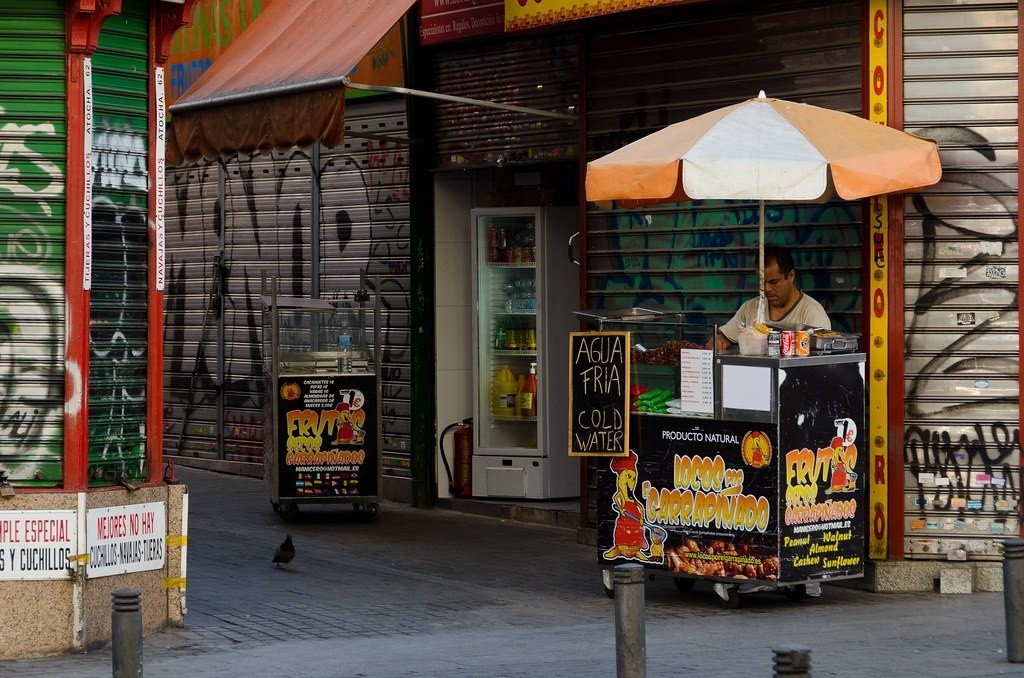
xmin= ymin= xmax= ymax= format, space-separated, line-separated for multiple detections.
xmin=766 ymin=330 xmax=810 ymax=356
xmin=489 ymin=227 xmax=535 ymax=266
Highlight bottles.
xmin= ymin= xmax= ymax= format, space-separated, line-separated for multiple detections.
xmin=514 ymin=220 xmax=535 ymax=246
xmin=495 ymin=318 xmax=528 ymax=350
xmin=337 ymin=320 xmax=352 ymax=374
xmin=503 ymin=274 xmax=536 ymax=310
xmin=493 ymin=362 xmax=537 ymax=418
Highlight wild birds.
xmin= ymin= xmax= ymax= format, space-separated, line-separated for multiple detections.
xmin=271 ymin=534 xmax=297 ymax=573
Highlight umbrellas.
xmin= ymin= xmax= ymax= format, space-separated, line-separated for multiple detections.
xmin=583 ymin=89 xmax=942 ymax=323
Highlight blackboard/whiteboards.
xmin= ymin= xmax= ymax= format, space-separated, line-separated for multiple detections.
xmin=567 ymin=331 xmax=631 ymax=458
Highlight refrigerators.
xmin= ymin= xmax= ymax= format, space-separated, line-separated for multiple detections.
xmin=470 ymin=205 xmax=580 ymax=500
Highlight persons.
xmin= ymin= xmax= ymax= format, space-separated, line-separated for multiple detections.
xmin=706 ymin=245 xmax=832 ymax=355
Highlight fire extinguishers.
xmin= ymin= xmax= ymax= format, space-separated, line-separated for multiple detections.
xmin=453 ymin=417 xmax=474 ymax=499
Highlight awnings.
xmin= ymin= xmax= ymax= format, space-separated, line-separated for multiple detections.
xmin=166 ymin=0 xmax=579 ymax=162
